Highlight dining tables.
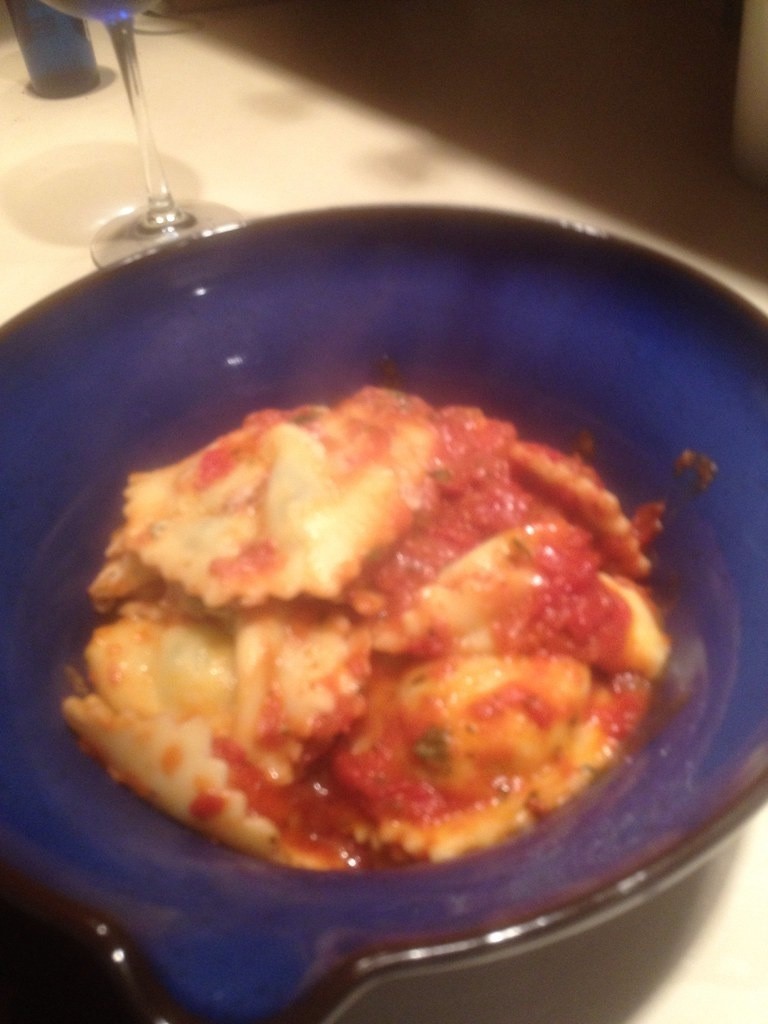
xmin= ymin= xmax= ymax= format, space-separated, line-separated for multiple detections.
xmin=1 ymin=0 xmax=767 ymax=1024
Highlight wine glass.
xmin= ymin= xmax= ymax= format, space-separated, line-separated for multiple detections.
xmin=44 ymin=0 xmax=248 ymax=270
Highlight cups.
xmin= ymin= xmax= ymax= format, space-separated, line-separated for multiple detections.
xmin=5 ymin=0 xmax=101 ymax=98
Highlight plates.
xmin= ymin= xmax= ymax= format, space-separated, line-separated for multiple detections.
xmin=1 ymin=202 xmax=768 ymax=1024
